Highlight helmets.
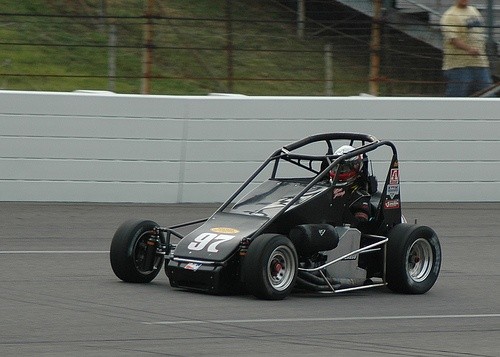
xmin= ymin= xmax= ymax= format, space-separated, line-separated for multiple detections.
xmin=329 ymin=144 xmax=364 ymax=187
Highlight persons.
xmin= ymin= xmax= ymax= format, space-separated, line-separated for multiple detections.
xmin=317 ymin=145 xmax=370 ymax=222
xmin=440 ymin=1 xmax=496 ymax=97
